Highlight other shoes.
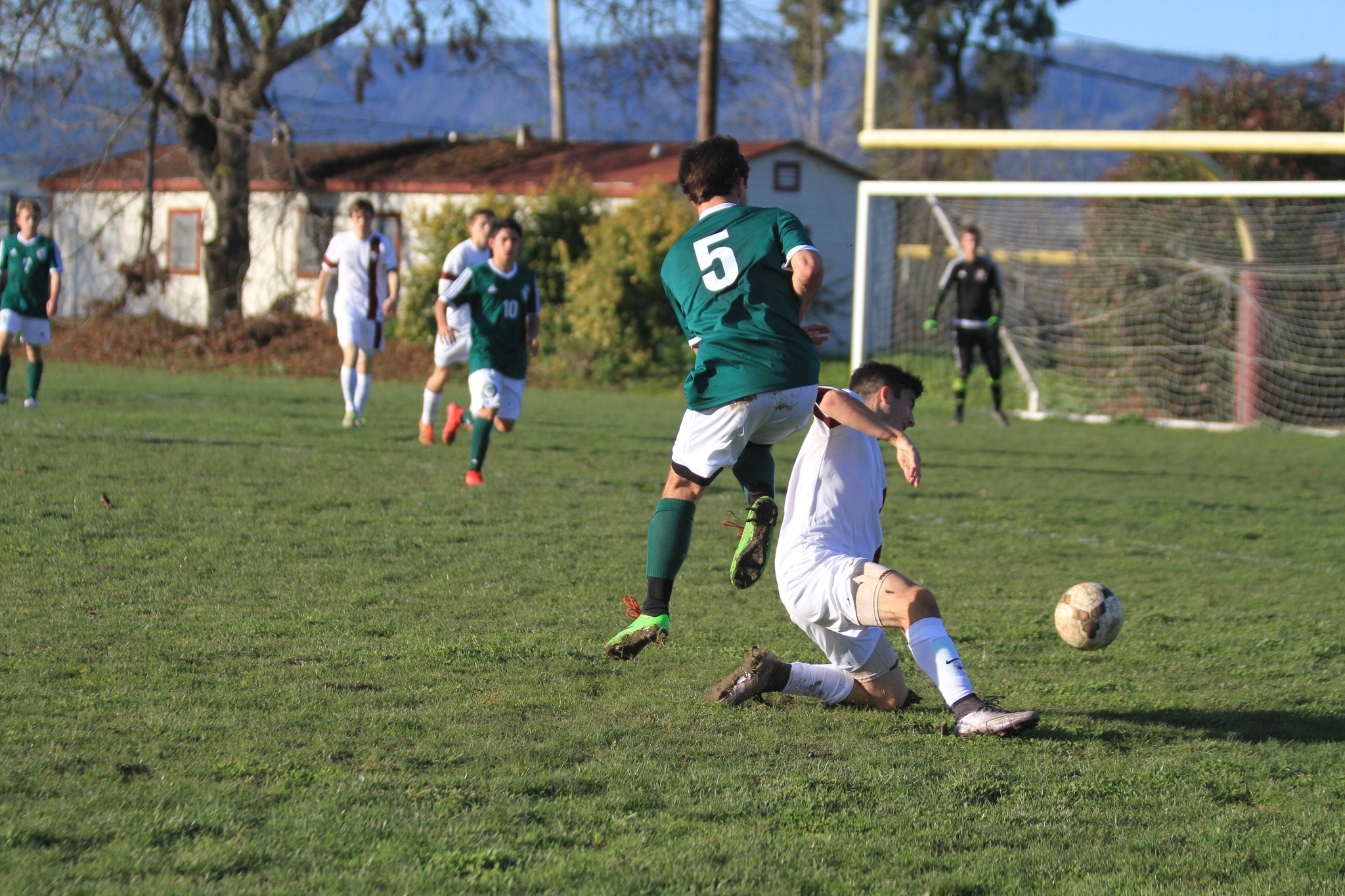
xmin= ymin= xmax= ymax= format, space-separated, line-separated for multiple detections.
xmin=354 ymin=416 xmax=367 ymax=429
xmin=951 ymin=409 xmax=964 ymax=425
xmin=24 ymin=398 xmax=37 ymax=409
xmin=0 ymin=392 xmax=8 ymax=404
xmin=342 ymin=410 xmax=358 ymax=430
xmin=994 ymin=410 xmax=1010 ymax=427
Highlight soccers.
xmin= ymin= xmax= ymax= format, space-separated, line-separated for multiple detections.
xmin=1055 ymin=581 xmax=1124 ymax=652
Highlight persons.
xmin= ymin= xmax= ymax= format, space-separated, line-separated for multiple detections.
xmin=922 ymin=224 xmax=1011 ymax=430
xmin=437 ymin=219 xmax=541 ymax=487
xmin=602 ymin=134 xmax=833 ymax=661
xmin=311 ymin=197 xmax=401 ymax=431
xmin=416 ymin=208 xmax=500 ymax=446
xmin=0 ymin=197 xmax=65 ymax=408
xmin=707 ymin=361 xmax=1040 ymax=738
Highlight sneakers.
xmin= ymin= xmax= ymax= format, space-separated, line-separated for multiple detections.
xmin=723 ymin=496 xmax=780 ymax=591
xmin=605 ymin=593 xmax=670 ymax=663
xmin=464 ymin=469 xmax=483 ymax=487
xmin=953 ymin=695 xmax=1041 ymax=739
xmin=442 ymin=402 xmax=464 ymax=445
xmin=418 ymin=418 xmax=436 ymax=445
xmin=701 ymin=646 xmax=781 ymax=712
